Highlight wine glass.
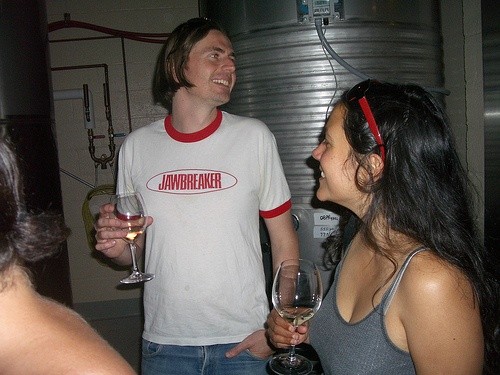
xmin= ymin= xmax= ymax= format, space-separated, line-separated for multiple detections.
xmin=265 ymin=257 xmax=322 ymax=375
xmin=109 ymin=190 xmax=155 ymax=284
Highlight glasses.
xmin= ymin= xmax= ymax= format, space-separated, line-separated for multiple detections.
xmin=171 ymin=17 xmax=219 ymax=56
xmin=344 ymin=79 xmax=387 ymax=163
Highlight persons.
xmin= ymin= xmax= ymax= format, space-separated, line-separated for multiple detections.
xmin=94 ymin=17 xmax=300 ymax=375
xmin=267 ymin=79 xmax=496 ymax=375
xmin=0 ymin=137 xmax=137 ymax=375
